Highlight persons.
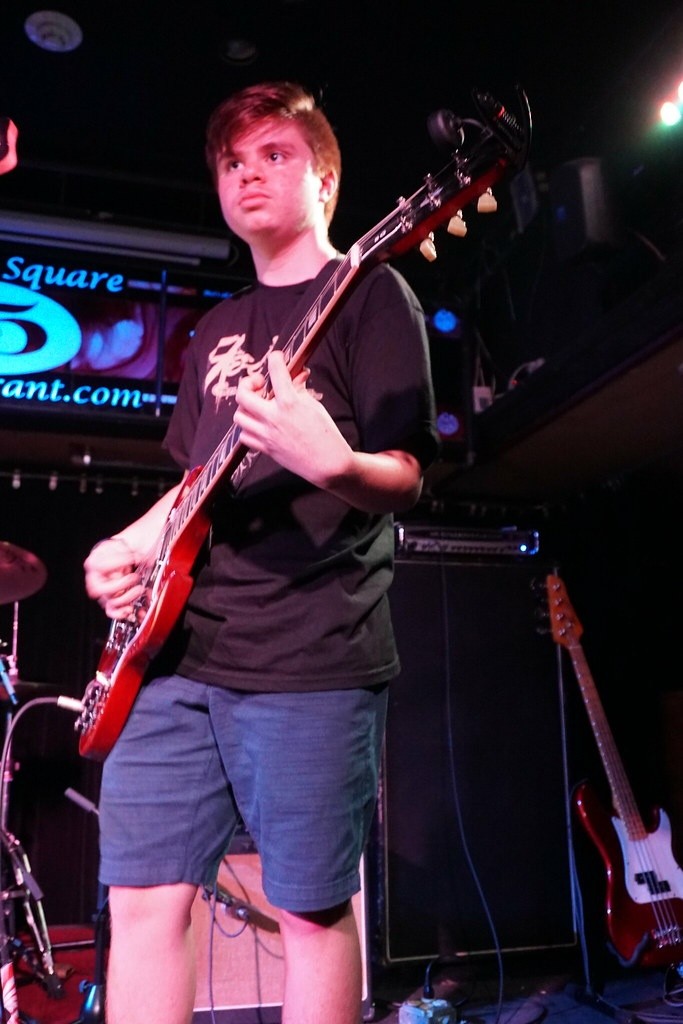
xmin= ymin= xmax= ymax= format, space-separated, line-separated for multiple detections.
xmin=84 ymin=82 xmax=439 ymax=1024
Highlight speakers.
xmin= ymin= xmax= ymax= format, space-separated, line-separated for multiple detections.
xmin=192 ymin=825 xmax=378 ymax=1024
xmin=372 ymin=552 xmax=581 ymax=977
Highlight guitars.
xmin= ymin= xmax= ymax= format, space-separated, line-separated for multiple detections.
xmin=546 ymin=571 xmax=683 ymax=972
xmin=71 ymin=84 xmax=535 ymax=760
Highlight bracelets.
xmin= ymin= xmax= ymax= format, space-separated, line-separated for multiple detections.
xmin=91 ymin=538 xmax=126 ymax=552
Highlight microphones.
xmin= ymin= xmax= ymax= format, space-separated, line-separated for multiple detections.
xmin=219 ymin=901 xmax=250 ymax=920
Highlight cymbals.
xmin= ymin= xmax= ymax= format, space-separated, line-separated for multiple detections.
xmin=0 ymin=678 xmax=77 ymax=706
xmin=0 ymin=540 xmax=48 ymax=605
